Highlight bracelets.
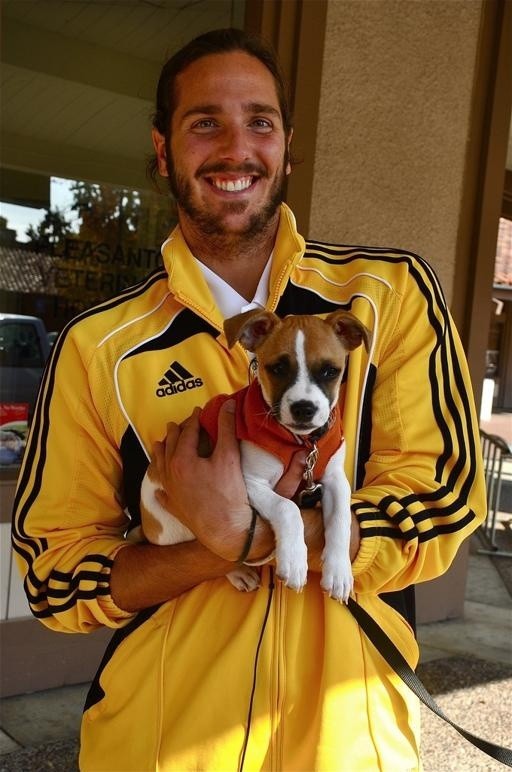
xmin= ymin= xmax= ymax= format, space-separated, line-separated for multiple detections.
xmin=236 ymin=549 xmax=282 ymax=567
xmin=235 ymin=507 xmax=258 ymax=565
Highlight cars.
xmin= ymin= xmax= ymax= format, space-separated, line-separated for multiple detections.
xmin=0 ymin=313 xmax=60 ymax=469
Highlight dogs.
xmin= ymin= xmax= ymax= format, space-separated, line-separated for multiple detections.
xmin=120 ymin=305 xmax=374 ymax=607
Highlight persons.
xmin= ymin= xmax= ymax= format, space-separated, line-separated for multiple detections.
xmin=6 ymin=25 xmax=491 ymax=771
